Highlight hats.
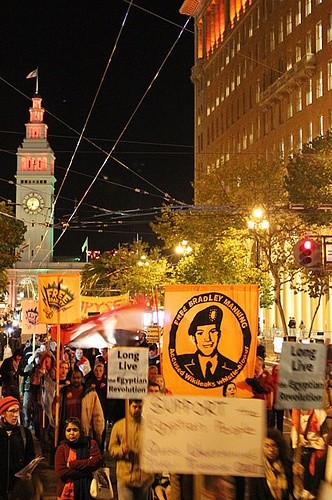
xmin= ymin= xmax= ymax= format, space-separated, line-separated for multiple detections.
xmin=0 ymin=395 xmax=20 ymax=415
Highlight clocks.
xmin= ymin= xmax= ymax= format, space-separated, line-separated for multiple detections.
xmin=23 ymin=193 xmax=44 ymax=216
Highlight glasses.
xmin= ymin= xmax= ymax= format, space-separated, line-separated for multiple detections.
xmin=263 ymin=444 xmax=281 ymax=450
xmin=5 ymin=409 xmax=21 ymax=413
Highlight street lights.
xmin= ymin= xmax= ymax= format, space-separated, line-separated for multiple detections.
xmin=249 ymin=208 xmax=269 ymax=266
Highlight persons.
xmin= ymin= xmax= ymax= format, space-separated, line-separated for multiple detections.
xmin=0 ymin=322 xmax=332 ymax=500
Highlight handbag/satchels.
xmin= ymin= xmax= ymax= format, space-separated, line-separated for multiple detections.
xmin=89 ymin=467 xmax=114 ymax=500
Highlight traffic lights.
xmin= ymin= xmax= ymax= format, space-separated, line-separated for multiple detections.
xmin=300 ymin=241 xmax=313 ymax=264
